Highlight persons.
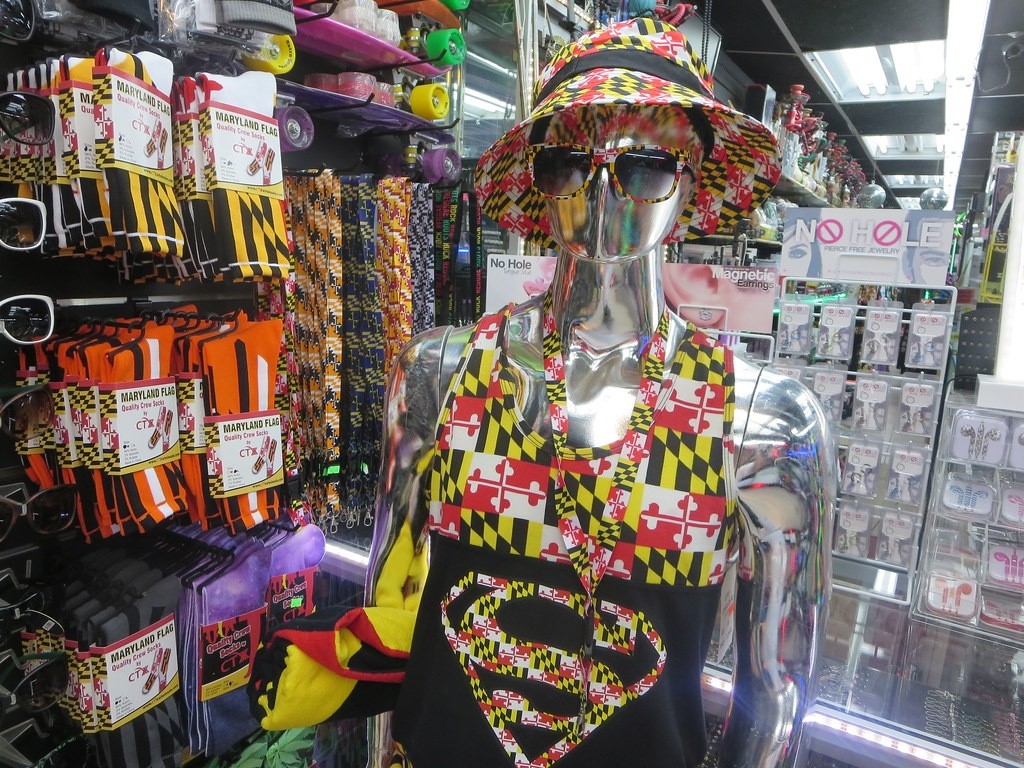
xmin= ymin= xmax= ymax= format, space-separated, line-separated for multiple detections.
xmin=365 ymin=18 xmax=834 ymax=768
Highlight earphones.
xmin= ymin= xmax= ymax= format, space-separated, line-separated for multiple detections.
xmin=925 ymin=410 xmax=1024 ymax=632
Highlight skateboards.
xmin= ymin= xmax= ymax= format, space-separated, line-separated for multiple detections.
xmin=240 ymin=0 xmax=472 ymax=120
xmin=181 ymin=48 xmax=462 ymax=186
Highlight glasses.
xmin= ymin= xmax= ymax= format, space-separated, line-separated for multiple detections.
xmin=0 ymin=651 xmax=69 ymax=718
xmin=29 ymin=734 xmax=92 ymax=768
xmin=0 ymin=294 xmax=56 ymax=345
xmin=0 ymin=92 xmax=56 ymax=145
xmin=0 ymin=384 xmax=55 ymax=440
xmin=0 ymin=0 xmax=36 ymax=43
xmin=0 ymin=198 xmax=48 ymax=251
xmin=0 ymin=482 xmax=82 ymax=545
xmin=526 ymin=133 xmax=701 ymax=203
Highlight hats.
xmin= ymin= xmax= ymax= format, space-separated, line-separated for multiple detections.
xmin=474 ymin=16 xmax=784 ymax=245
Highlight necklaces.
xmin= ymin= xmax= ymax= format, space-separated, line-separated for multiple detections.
xmin=541 ymin=283 xmax=669 ymax=735
